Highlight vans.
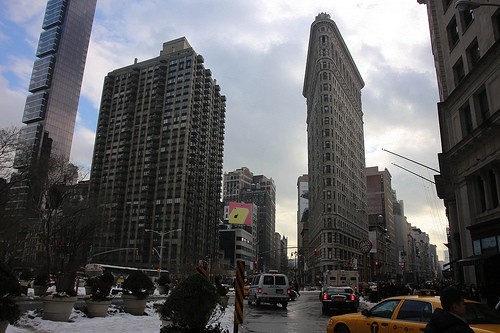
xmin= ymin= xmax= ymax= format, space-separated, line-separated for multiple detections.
xmin=247 ymin=269 xmax=292 ymax=309
xmin=242 ymin=275 xmax=252 ymax=297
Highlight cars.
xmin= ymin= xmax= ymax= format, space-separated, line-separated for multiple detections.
xmin=321 ymin=287 xmax=358 ymax=315
xmin=326 ymin=294 xmax=500 ymax=333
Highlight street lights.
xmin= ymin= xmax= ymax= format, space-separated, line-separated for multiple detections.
xmin=291 ymin=250 xmax=301 ymax=281
xmin=144 ymin=228 xmax=181 ymax=283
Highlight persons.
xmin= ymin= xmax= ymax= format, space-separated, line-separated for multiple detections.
xmin=380 ymin=279 xmax=409 ymax=300
xmin=424 ymin=286 xmax=475 ymax=333
xmin=294 ymin=281 xmax=301 ymax=296
xmin=436 ymin=278 xmax=490 ymax=302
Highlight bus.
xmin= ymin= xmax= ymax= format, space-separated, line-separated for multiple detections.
xmin=83 ymin=263 xmax=169 ymax=289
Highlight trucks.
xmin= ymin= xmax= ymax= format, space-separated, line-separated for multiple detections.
xmin=321 ymin=270 xmax=359 ymax=293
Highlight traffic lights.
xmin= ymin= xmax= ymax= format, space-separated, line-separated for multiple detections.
xmin=375 ymin=262 xmax=378 ymax=269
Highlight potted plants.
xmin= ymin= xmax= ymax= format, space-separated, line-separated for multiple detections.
xmin=43 ymin=276 xmax=79 ymax=322
xmin=83 ymin=275 xmax=95 ymax=294
xmin=214 ymin=275 xmax=231 ymax=308
xmin=33 ymin=279 xmax=48 ymax=295
xmin=157 ymin=275 xmax=172 ymax=294
xmin=121 ymin=270 xmax=155 ymax=315
xmin=84 ymin=271 xmax=114 ymax=318
xmin=0 ymin=262 xmax=26 ymax=333
